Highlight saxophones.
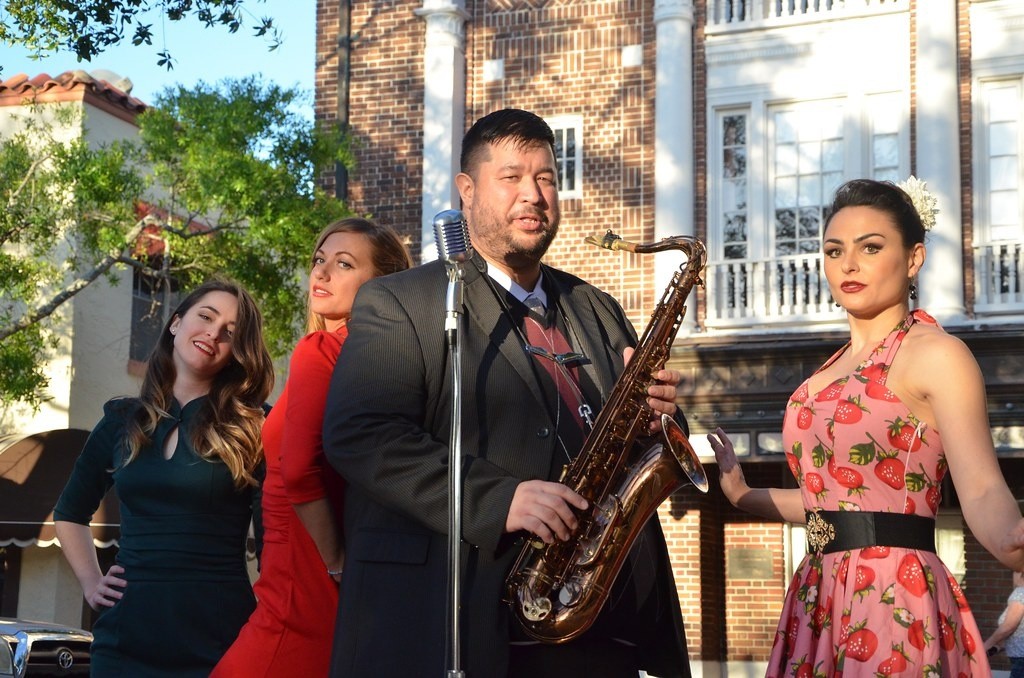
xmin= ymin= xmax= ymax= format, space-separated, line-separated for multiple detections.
xmin=503 ymin=225 xmax=712 ymax=645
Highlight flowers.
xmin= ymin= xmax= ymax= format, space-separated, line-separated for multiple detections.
xmin=895 ymin=175 xmax=941 ymax=232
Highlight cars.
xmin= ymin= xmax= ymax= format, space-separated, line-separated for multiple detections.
xmin=0 ymin=616 xmax=97 ymax=678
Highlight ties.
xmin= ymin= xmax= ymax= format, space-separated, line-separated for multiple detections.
xmin=523 ymin=297 xmax=546 ymax=317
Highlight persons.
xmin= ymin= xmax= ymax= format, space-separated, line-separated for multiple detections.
xmin=707 ymin=177 xmax=1024 ymax=678
xmin=313 ymin=108 xmax=691 ymax=678
xmin=981 ymin=571 xmax=1024 ymax=678
xmin=208 ymin=217 xmax=412 ymax=678
xmin=54 ymin=281 xmax=277 ymax=678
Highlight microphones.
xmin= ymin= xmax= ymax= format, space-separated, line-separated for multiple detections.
xmin=433 ymin=210 xmax=474 ymax=349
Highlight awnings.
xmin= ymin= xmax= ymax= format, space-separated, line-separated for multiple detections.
xmin=0 ymin=426 xmax=119 ymax=550
